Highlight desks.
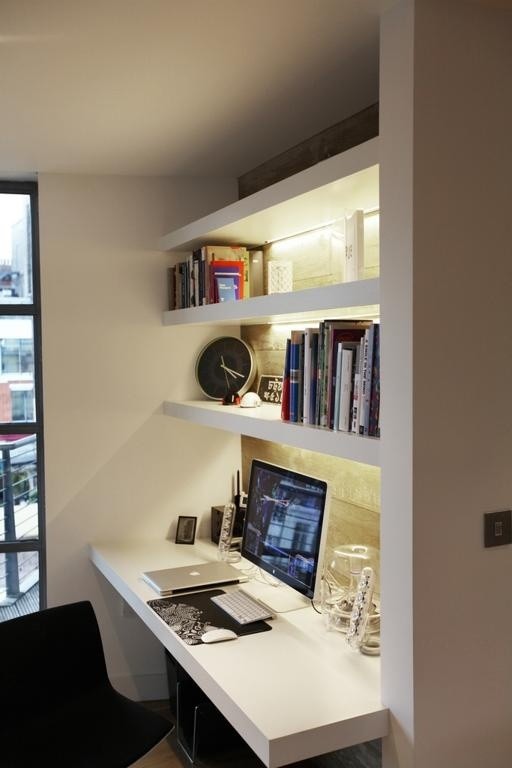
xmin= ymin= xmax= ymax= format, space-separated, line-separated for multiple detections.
xmin=87 ymin=536 xmax=388 ymax=768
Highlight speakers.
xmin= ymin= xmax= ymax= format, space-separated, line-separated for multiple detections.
xmin=218 ymin=503 xmax=240 ymax=563
xmin=346 ymin=566 xmax=377 ymax=648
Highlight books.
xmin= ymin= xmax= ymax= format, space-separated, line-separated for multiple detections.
xmin=168 ymin=244 xmax=264 ymax=310
xmin=281 ymin=318 xmax=380 ymax=438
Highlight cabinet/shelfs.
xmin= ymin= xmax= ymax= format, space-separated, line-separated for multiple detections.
xmin=148 ymin=136 xmax=380 ymax=471
xmin=165 ymin=648 xmax=325 ymax=767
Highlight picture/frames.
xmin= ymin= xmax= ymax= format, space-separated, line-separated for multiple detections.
xmin=174 ymin=515 xmax=196 ymax=545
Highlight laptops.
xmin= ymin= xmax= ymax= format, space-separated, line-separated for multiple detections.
xmin=142 ymin=560 xmax=248 ymax=596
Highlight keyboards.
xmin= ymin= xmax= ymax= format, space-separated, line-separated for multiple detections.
xmin=210 ymin=591 xmax=272 ymax=625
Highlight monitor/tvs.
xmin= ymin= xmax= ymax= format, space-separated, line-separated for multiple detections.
xmin=240 ymin=458 xmax=332 ymax=613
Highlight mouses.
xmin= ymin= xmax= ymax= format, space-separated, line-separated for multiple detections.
xmin=201 ymin=629 xmax=237 ymax=644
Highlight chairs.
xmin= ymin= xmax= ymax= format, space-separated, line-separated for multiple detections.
xmin=0 ymin=601 xmax=176 ymax=768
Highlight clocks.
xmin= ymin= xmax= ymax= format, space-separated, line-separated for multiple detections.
xmin=190 ymin=333 xmax=258 ymax=404
